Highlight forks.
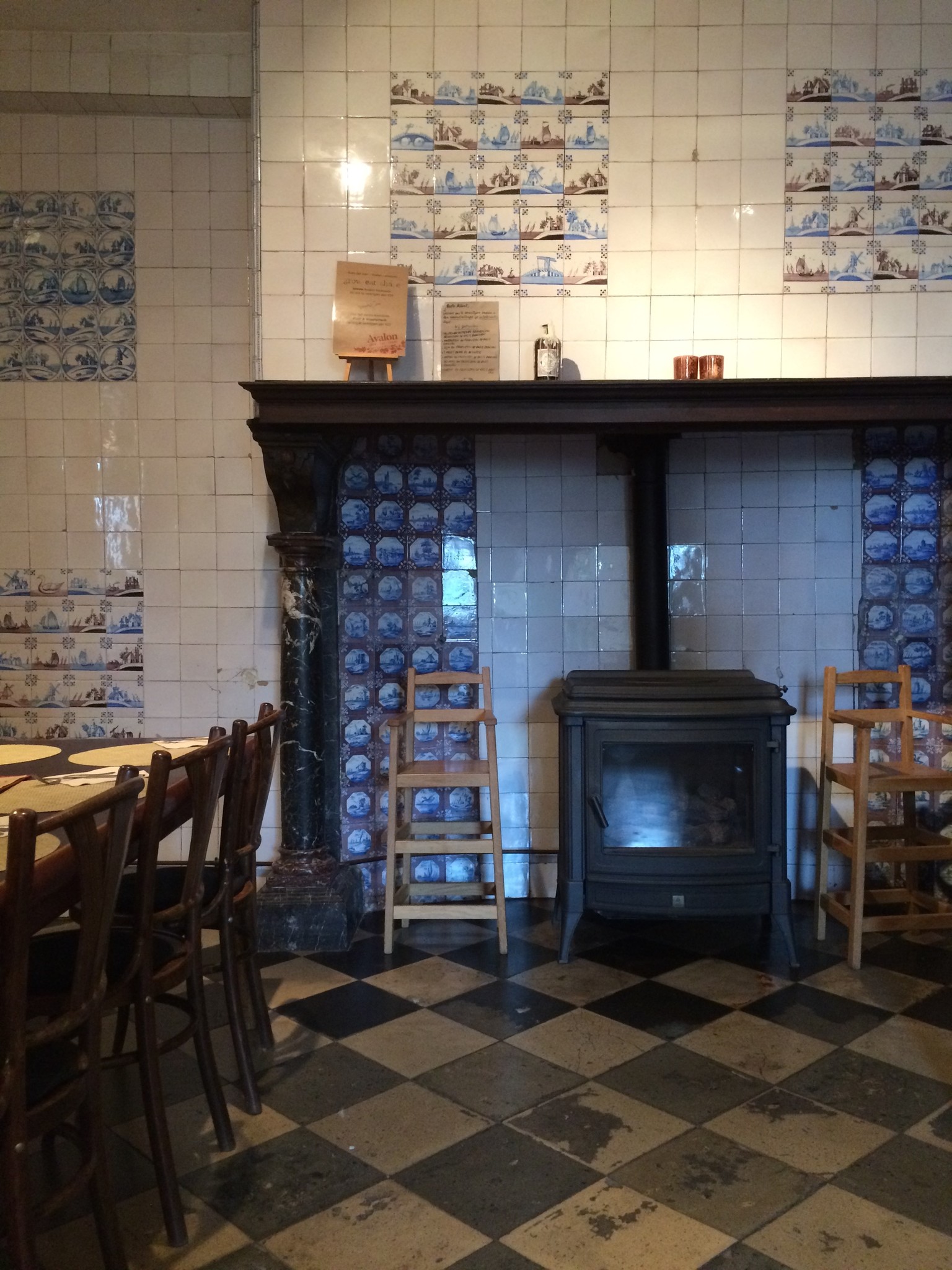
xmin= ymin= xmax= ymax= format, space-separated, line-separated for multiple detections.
xmin=155 ymin=733 xmax=209 ymax=743
xmin=29 ymin=772 xmax=144 ymax=786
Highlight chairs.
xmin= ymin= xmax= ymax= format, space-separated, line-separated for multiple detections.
xmin=816 ymin=665 xmax=951 ymax=968
xmin=385 ymin=665 xmax=511 ymax=956
xmin=1 ymin=700 xmax=291 ymax=1268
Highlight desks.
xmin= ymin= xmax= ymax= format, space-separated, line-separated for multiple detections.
xmin=0 ymin=733 xmax=262 ymax=983
